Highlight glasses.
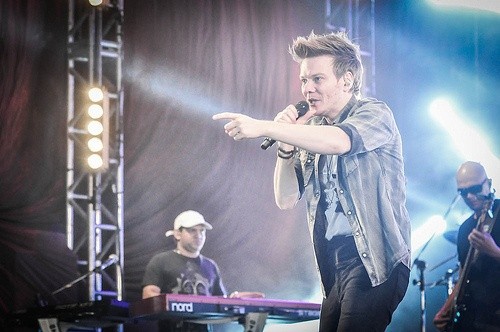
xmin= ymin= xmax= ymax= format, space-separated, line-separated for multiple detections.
xmin=457 ymin=178 xmax=486 ymax=196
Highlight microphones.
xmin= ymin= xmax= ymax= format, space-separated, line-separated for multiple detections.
xmin=97 ymin=254 xmax=118 ymax=272
xmin=445 ymin=262 xmax=460 ymax=278
xmin=261 ymin=101 xmax=309 ymax=150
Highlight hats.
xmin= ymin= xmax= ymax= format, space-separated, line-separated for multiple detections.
xmin=165 ymin=210 xmax=213 ymax=237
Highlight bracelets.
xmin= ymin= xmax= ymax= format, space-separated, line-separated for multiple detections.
xmin=229 ymin=291 xmax=239 ymax=298
xmin=277 ymin=146 xmax=298 ymax=160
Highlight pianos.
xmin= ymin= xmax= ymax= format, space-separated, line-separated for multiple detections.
xmin=129 ymin=293 xmax=322 ymax=321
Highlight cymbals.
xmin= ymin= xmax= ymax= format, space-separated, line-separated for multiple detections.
xmin=443 ymin=230 xmax=459 ymax=245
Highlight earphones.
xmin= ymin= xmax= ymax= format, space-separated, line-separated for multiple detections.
xmin=344 ymin=78 xmax=350 ymax=86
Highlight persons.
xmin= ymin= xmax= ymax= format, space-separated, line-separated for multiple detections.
xmin=210 ymin=30 xmax=412 ymax=332
xmin=432 ymin=160 xmax=500 ymax=332
xmin=141 ymin=210 xmax=265 ymax=332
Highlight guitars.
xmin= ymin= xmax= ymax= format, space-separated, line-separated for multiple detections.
xmin=436 ymin=192 xmax=494 ymax=323
